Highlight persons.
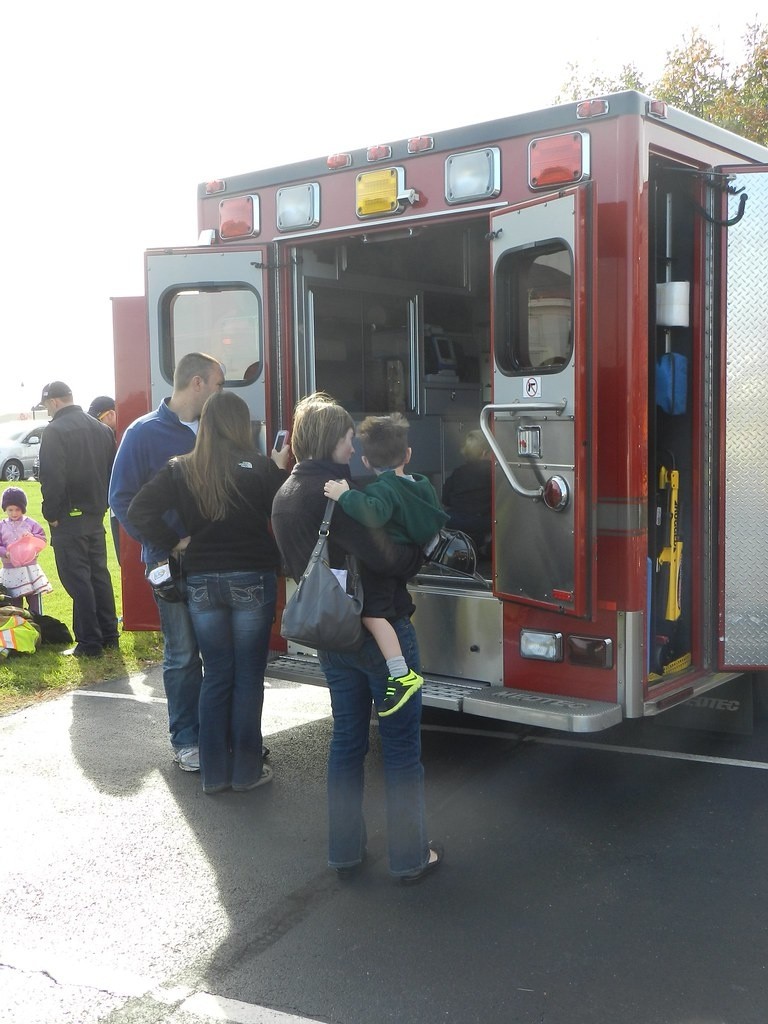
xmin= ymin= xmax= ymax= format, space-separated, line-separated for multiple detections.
xmin=31 ymin=380 xmax=116 ymax=658
xmin=270 ymin=391 xmax=443 ymax=885
xmin=440 ymin=429 xmax=493 ymax=561
xmin=108 ymin=351 xmax=291 ymax=795
xmin=0 ymin=487 xmax=54 ymax=616
xmin=322 ymin=412 xmax=452 ymax=718
xmin=87 ymin=396 xmax=119 ymax=566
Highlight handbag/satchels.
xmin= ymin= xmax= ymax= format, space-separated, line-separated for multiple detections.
xmin=279 ymin=479 xmax=366 ymax=651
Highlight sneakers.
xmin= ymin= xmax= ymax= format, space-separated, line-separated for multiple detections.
xmin=174 ymin=746 xmax=200 ymax=772
xmin=379 ymin=669 xmax=424 ymax=716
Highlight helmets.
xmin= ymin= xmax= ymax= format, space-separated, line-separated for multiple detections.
xmin=426 ymin=528 xmax=478 ymax=577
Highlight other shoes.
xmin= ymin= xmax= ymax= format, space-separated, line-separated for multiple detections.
xmin=232 ymin=764 xmax=271 ymax=791
xmin=62 ymin=646 xmax=92 ymax=658
xmin=202 ymin=781 xmax=231 ymax=794
xmin=336 ymin=848 xmax=377 ymax=873
xmin=402 ymin=840 xmax=443 ymax=884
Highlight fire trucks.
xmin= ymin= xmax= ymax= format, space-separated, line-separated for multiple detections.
xmin=108 ymin=89 xmax=767 ymax=735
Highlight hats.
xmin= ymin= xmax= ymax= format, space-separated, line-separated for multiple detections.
xmin=37 ymin=381 xmax=71 ymax=405
xmin=87 ymin=397 xmax=114 ymax=420
xmin=2 ymin=488 xmax=27 ymax=514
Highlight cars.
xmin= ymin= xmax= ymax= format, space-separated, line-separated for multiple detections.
xmin=0 ymin=421 xmax=49 ymax=482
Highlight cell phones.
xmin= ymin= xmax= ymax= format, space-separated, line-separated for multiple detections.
xmin=273 ymin=430 xmax=289 ymax=452
